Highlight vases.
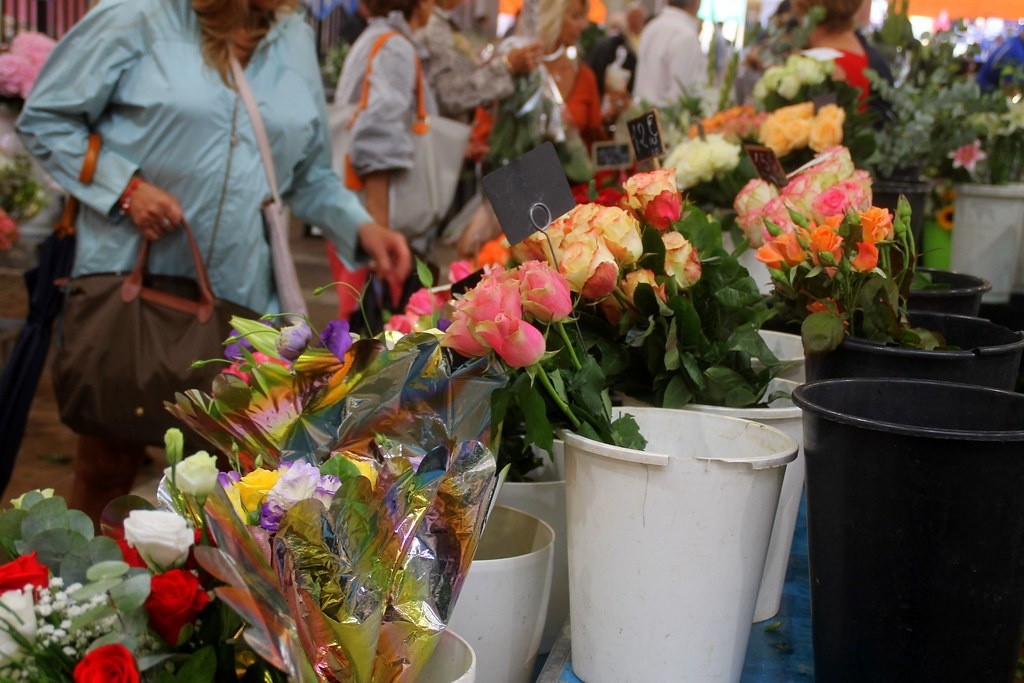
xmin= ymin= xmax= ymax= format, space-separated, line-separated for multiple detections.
xmin=897 ymin=268 xmax=992 ymax=317
xmin=951 ymin=185 xmax=1024 ymax=302
xmin=612 ymin=376 xmax=806 ymax=624
xmin=493 ymin=435 xmax=570 ymax=656
xmin=792 ymin=378 xmax=1024 ymax=683
xmin=922 ymin=211 xmax=953 ymax=271
xmin=448 ymin=502 xmax=555 ymax=683
xmin=288 ymin=628 xmax=477 ymax=683
xmin=870 ymin=178 xmax=933 ymax=278
xmin=840 ymin=309 xmax=1024 ymax=392
xmin=733 ymin=327 xmax=806 ymax=385
xmin=562 ymin=405 xmax=800 ymax=683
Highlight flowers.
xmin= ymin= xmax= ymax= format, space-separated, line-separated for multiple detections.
xmin=0 ymin=48 xmax=1024 ymax=683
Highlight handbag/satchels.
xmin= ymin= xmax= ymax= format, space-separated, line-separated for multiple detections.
xmin=53 ymin=214 xmax=290 ymax=466
xmin=326 ymin=30 xmax=471 ymax=234
xmin=261 ymin=200 xmax=339 ymax=348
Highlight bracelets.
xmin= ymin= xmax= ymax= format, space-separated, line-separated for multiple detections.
xmin=118 ymin=178 xmax=148 ymax=214
xmin=501 ymin=55 xmax=514 ymax=73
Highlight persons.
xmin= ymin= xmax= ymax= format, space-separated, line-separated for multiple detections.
xmin=326 ymin=0 xmax=894 ymax=323
xmin=14 ymin=0 xmax=411 ymax=533
xmin=975 ymin=30 xmax=1024 ymax=93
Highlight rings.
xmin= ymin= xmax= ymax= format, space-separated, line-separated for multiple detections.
xmin=162 ymin=221 xmax=172 ymax=227
xmin=616 ymin=100 xmax=624 ymax=108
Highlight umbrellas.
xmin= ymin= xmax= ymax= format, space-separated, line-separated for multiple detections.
xmin=0 ymin=129 xmax=103 ymax=509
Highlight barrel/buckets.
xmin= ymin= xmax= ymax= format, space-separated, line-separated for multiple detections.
xmin=415 ymin=177 xmax=1024 ymax=683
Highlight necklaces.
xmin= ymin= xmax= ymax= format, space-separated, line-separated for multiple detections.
xmin=548 ymin=66 xmax=566 ymax=84
